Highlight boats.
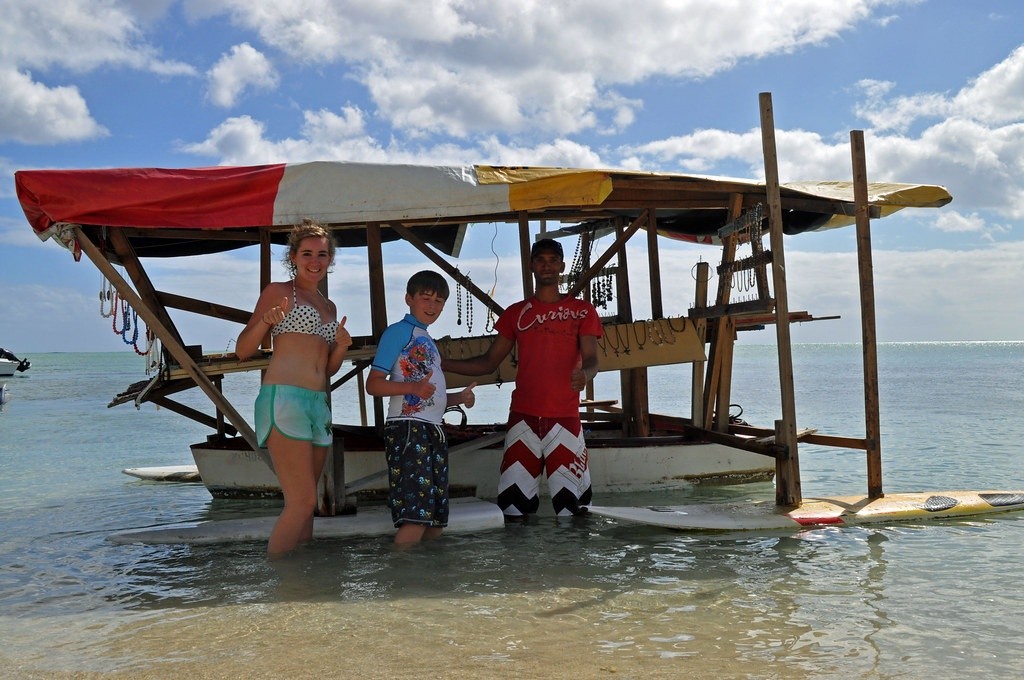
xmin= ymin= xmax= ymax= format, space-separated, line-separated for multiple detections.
xmin=0 ymin=348 xmax=32 ymax=377
xmin=15 ymin=162 xmax=955 ymax=507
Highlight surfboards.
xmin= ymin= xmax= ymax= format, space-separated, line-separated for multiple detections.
xmin=577 ymin=489 xmax=1023 ymax=529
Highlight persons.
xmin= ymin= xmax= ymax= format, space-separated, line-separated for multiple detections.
xmin=440 ymin=239 xmax=605 ymax=516
xmin=365 ymin=269 xmax=477 ymax=545
xmin=235 ymin=217 xmax=352 ymax=553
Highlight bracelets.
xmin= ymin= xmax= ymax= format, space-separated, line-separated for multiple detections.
xmin=262 ymin=317 xmax=269 ymax=325
